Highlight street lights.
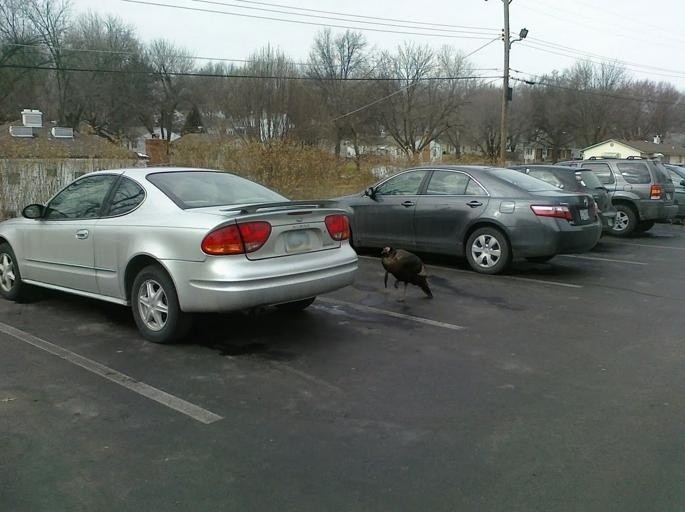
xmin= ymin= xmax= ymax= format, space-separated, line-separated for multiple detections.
xmin=501 ymin=28 xmax=528 ymax=167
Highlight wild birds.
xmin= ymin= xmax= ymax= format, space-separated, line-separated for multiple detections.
xmin=379 ymin=245 xmax=434 ymax=303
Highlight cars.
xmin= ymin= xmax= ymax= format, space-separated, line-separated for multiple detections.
xmin=0 ymin=166 xmax=358 ymax=342
